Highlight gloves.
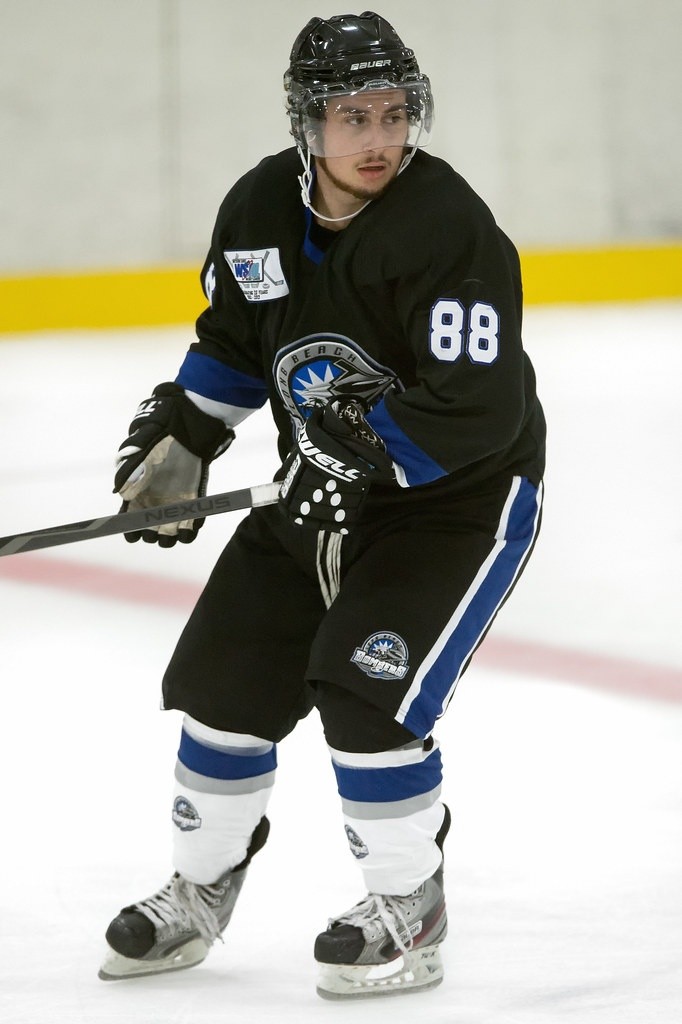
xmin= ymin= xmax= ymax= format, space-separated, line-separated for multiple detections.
xmin=115 ymin=383 xmax=234 ymax=547
xmin=275 ymin=397 xmax=393 ymax=533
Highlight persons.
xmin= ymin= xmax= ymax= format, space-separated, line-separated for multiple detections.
xmin=76 ymin=5 xmax=558 ymax=971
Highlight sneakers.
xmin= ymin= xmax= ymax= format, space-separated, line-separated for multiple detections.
xmin=314 ymin=864 xmax=448 ymax=1001
xmin=99 ymin=867 xmax=248 ymax=978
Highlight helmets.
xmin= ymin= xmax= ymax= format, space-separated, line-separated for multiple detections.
xmin=288 ymin=14 xmax=423 ymax=118
